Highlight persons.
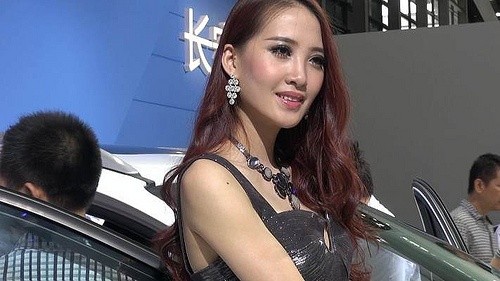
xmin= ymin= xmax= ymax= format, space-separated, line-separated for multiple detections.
xmin=446 ymin=152 xmax=500 ymax=271
xmin=0 ymin=109 xmax=139 ymax=281
xmin=158 ymin=0 xmax=422 ymax=281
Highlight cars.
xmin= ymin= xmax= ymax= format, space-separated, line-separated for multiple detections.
xmin=0 ymin=145 xmax=500 ymax=281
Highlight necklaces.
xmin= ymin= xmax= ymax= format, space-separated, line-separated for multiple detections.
xmin=226 ymin=134 xmax=301 ymax=208
